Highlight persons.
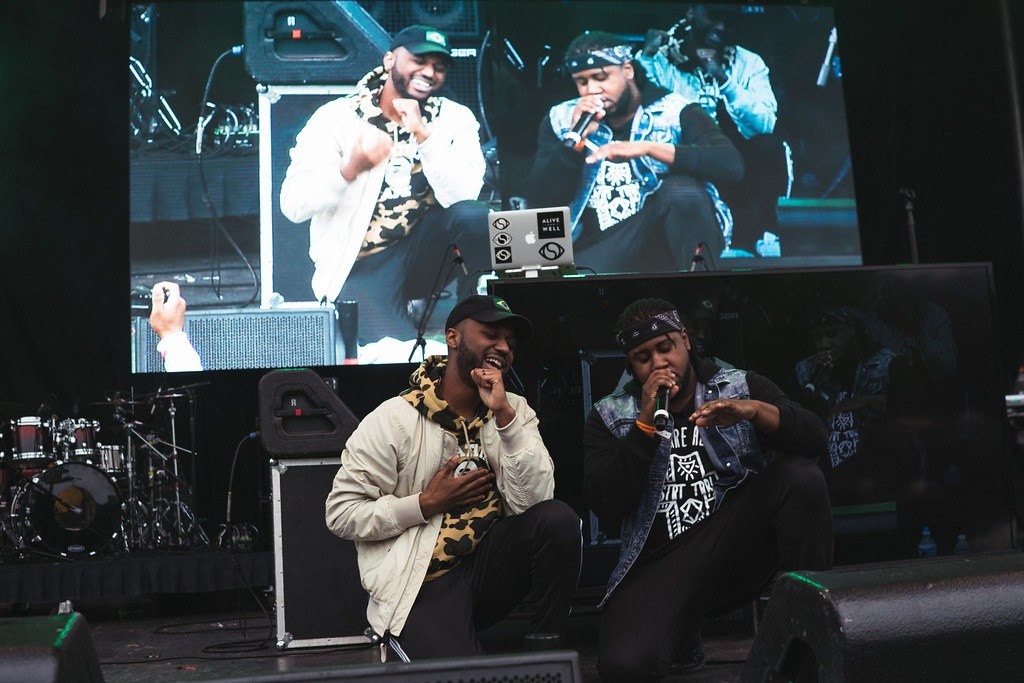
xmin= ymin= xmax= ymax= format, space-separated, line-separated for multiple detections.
xmin=149 ymin=280 xmax=203 ymax=372
xmin=524 ymin=30 xmax=744 ymax=274
xmin=634 ymin=12 xmax=793 ymax=258
xmin=582 ymin=298 xmax=835 ymax=683
xmin=279 ymin=23 xmax=511 ymax=346
xmin=680 ymin=272 xmax=979 ymax=542
xmin=326 ymin=295 xmax=582 ymax=661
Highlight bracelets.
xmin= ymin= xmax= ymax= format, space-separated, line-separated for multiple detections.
xmin=635 ymin=420 xmax=656 ymax=434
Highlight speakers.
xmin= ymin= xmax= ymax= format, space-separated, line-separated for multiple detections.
xmin=739 ymin=546 xmax=1024 ymax=683
xmin=255 ymin=367 xmax=361 ymax=456
xmin=355 ymin=0 xmax=501 ymax=210
xmin=0 ymin=612 xmax=104 ymax=683
xmin=130 ymin=305 xmax=336 ymax=374
xmin=242 ymin=0 xmax=395 ymax=84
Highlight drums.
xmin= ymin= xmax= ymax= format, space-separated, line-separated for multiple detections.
xmin=51 ymin=420 xmax=100 ymax=461
xmin=8 ymin=414 xmax=56 ymax=466
xmin=10 ymin=461 xmax=124 ymax=559
xmin=98 ymin=444 xmax=127 ymax=474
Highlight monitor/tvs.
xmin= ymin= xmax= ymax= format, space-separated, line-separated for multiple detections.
xmin=487 ymin=206 xmax=578 ymax=269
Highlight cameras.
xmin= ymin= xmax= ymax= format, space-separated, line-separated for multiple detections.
xmin=130 ymin=284 xmax=169 ymax=316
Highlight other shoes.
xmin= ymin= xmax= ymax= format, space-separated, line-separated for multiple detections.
xmin=752 ymin=231 xmax=780 ymax=257
xmin=669 ymin=627 xmax=705 ymax=673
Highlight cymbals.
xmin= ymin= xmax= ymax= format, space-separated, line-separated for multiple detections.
xmin=92 ymin=398 xmax=146 ymax=405
xmin=154 ymin=393 xmax=188 ymax=399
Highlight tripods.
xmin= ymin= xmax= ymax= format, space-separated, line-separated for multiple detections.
xmin=111 ymin=400 xmax=211 ymax=552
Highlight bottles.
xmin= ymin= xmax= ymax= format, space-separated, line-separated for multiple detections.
xmin=1013 ymin=367 xmax=1024 ymax=396
xmin=954 ymin=535 xmax=972 ymax=556
xmin=917 ymin=527 xmax=937 ymax=557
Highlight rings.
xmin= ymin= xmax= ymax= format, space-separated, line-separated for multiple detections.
xmin=482 ymin=369 xmax=487 ymax=376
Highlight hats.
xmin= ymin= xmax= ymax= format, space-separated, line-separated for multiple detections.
xmin=389 ymin=25 xmax=451 ymax=56
xmin=666 ymin=293 xmax=719 ymax=322
xmin=444 ymin=295 xmax=532 ymax=344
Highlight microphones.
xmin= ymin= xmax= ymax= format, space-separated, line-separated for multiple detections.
xmin=801 ymin=355 xmax=832 ymax=398
xmin=653 ymin=385 xmax=670 ymax=431
xmin=561 ymin=111 xmax=595 ymax=150
xmin=451 ymin=242 xmax=469 ymax=277
xmin=689 ymin=245 xmax=702 ymax=271
